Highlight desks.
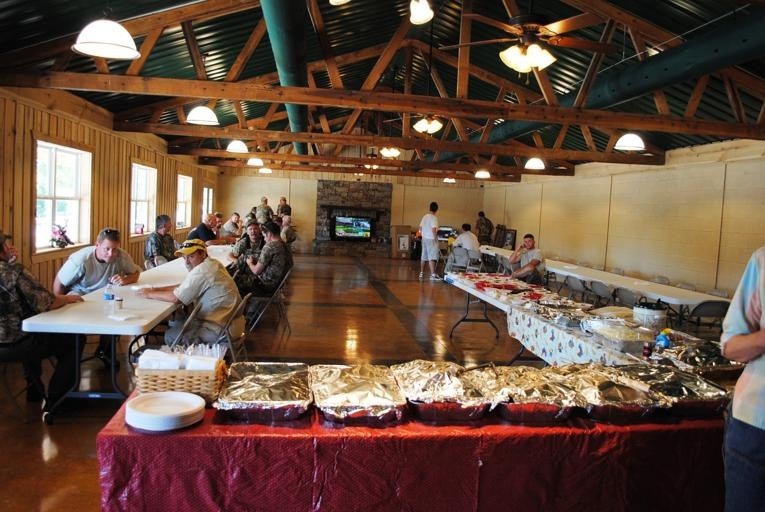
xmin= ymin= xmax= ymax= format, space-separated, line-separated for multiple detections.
xmin=89 ymin=372 xmax=727 ymax=512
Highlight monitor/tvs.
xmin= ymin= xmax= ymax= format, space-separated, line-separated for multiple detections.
xmin=332 ymin=215 xmax=372 ymax=240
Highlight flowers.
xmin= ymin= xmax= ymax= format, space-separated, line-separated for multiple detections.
xmin=49 ymin=223 xmax=74 ymax=246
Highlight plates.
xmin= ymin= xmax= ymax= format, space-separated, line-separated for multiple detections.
xmin=124 ymin=391 xmax=208 ymax=435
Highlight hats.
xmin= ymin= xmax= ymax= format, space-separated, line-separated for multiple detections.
xmin=174 ymin=239 xmax=207 ymax=256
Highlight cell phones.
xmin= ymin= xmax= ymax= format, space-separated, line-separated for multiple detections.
xmin=521 ymin=243 xmax=525 ymax=248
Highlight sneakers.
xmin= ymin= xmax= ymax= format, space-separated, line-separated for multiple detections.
xmin=430 ymin=275 xmax=444 ymax=281
xmin=418 ymin=272 xmax=423 ymax=277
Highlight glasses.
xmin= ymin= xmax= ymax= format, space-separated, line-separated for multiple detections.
xmin=180 ymin=243 xmax=204 ymax=248
xmin=103 ymin=229 xmax=118 ymax=235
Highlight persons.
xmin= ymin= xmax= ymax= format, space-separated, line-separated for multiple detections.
xmin=475 ymin=210 xmax=496 ymax=241
xmin=453 ymin=222 xmax=479 ymax=263
xmin=507 ymin=234 xmax=548 ymax=279
xmin=1 ymin=231 xmax=83 ymax=402
xmin=716 ymin=237 xmax=765 ymax=512
xmin=55 ymin=225 xmax=142 ymax=368
xmin=418 ymin=200 xmax=443 ymax=282
xmin=133 ymin=237 xmax=246 ymax=345
xmin=184 ymin=197 xmax=297 ymax=297
xmin=142 ymin=214 xmax=182 ymax=270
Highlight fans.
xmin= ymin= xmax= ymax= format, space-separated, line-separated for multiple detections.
xmin=435 ymin=1 xmax=619 ymax=56
xmin=382 ymin=18 xmax=445 ymax=122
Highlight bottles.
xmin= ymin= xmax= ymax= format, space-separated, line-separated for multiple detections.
xmin=103 ymin=281 xmax=115 ymax=318
xmin=113 ymin=297 xmax=126 ymax=323
xmin=642 ymin=341 xmax=652 ymax=361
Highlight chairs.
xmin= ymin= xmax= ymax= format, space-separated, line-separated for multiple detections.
xmin=437 ymin=242 xmax=737 ymax=372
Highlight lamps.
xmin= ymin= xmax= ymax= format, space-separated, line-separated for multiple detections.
xmin=412 ymin=117 xmax=442 ymax=135
xmin=523 ymin=155 xmax=545 ymax=170
xmin=67 ymin=2 xmax=140 ymax=64
xmin=474 ymin=168 xmax=492 ymax=180
xmin=613 ymin=129 xmax=647 ymax=151
xmin=405 ymin=0 xmax=434 ymax=27
xmin=182 ymin=99 xmax=274 ymax=174
xmin=496 ymin=43 xmax=559 ymax=75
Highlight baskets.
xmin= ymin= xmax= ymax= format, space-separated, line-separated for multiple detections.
xmin=135 ymin=359 xmax=224 ymax=406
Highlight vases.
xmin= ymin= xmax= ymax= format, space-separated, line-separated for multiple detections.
xmin=59 ymin=244 xmax=65 ymax=249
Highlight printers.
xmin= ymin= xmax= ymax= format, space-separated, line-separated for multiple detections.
xmin=438 ymin=225 xmax=452 ymax=240
